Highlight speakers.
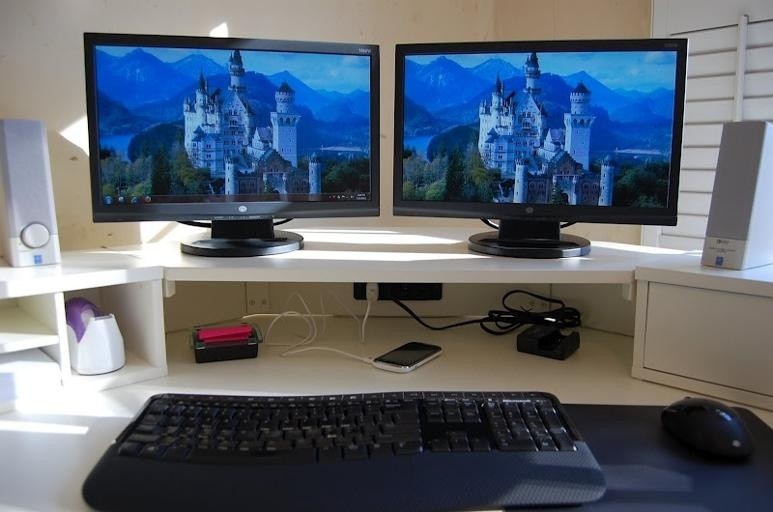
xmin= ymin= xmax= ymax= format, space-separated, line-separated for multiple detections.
xmin=702 ymin=120 xmax=773 ymax=272
xmin=0 ymin=118 xmax=62 ymax=267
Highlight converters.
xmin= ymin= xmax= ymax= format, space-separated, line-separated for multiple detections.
xmin=366 ymin=283 xmax=379 ymax=302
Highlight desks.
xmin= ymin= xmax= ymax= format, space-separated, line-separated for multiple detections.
xmin=1 ymin=225 xmax=773 ymax=510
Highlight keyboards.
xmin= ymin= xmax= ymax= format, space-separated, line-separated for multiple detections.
xmin=83 ymin=391 xmax=607 ymax=512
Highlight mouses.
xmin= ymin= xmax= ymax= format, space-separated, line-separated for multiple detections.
xmin=661 ymin=395 xmax=755 ymax=458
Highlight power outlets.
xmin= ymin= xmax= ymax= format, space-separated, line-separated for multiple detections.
xmin=353 ymin=284 xmax=444 ymax=301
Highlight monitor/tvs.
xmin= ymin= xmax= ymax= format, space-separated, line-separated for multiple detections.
xmin=392 ymin=38 xmax=688 ymax=259
xmin=83 ymin=32 xmax=380 ymax=257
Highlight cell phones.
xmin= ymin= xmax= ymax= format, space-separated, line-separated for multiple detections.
xmin=371 ymin=341 xmax=443 ymax=373
